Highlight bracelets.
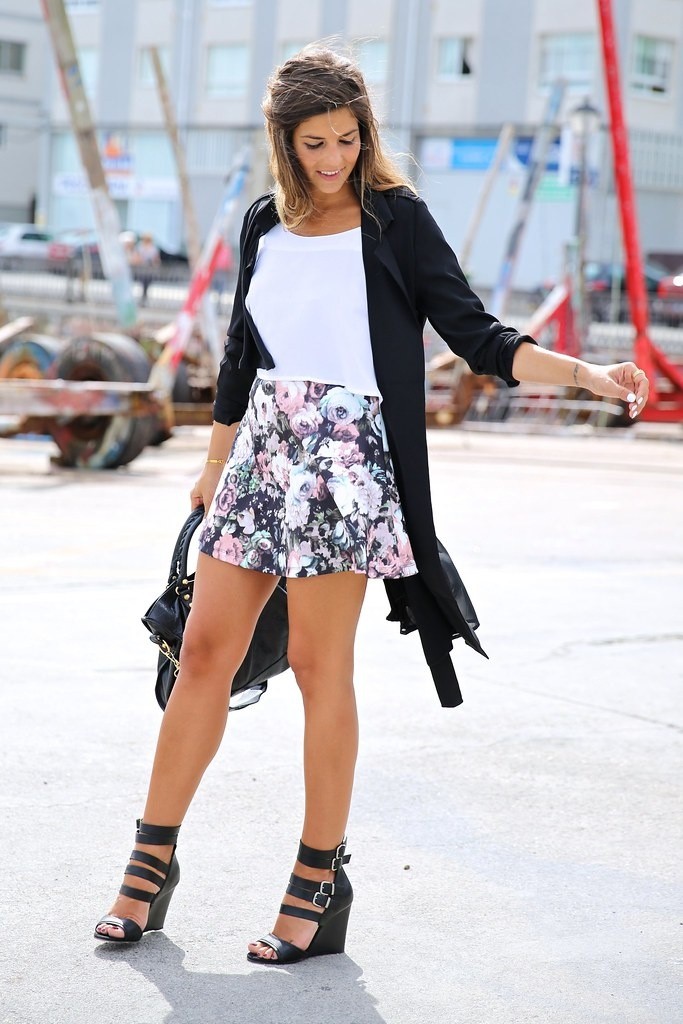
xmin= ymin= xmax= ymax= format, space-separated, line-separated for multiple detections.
xmin=203 ymin=459 xmax=225 ymax=465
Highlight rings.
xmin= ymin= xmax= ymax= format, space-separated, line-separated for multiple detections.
xmin=633 ymin=368 xmax=645 ymax=380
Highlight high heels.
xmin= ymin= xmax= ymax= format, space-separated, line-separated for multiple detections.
xmin=246 ymin=834 xmax=354 ymax=965
xmin=93 ymin=818 xmax=182 ymax=944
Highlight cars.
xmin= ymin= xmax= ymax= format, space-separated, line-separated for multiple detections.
xmin=0 ymin=217 xmax=164 ymax=285
xmin=543 ymin=256 xmax=683 ymax=324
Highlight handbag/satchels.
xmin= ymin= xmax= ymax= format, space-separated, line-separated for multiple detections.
xmin=141 ymin=502 xmax=291 ymax=713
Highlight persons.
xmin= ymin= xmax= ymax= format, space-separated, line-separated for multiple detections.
xmin=93 ymin=45 xmax=649 ymax=964
xmin=65 ymin=231 xmax=162 ymax=303
